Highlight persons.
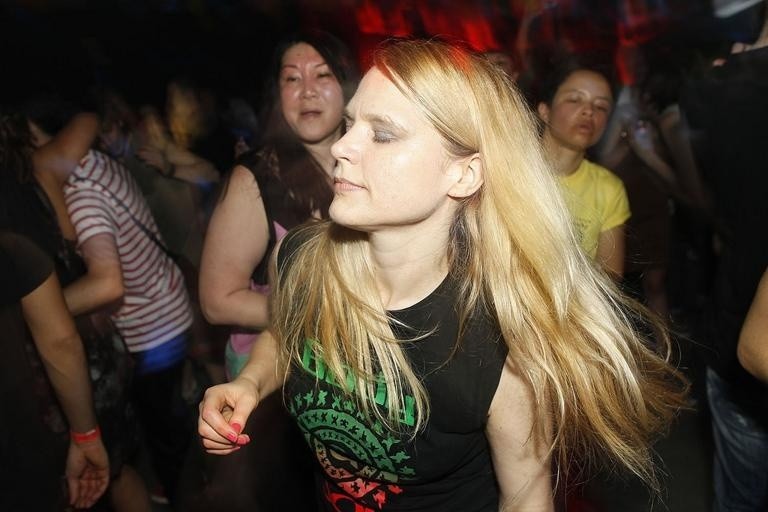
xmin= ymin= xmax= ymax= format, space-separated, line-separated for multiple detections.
xmin=125 ymin=75 xmax=222 ymax=186
xmin=1 ymin=107 xmax=167 ymax=511
xmin=619 ymin=98 xmax=723 ymax=363
xmin=190 ymin=37 xmax=691 ymax=512
xmin=534 ymin=60 xmax=630 ymax=287
xmin=2 ymin=230 xmax=110 ymax=512
xmin=704 ymin=55 xmax=766 ymax=511
xmin=25 ymin=103 xmax=188 ymax=381
xmin=197 ymin=23 xmax=372 ymax=511
